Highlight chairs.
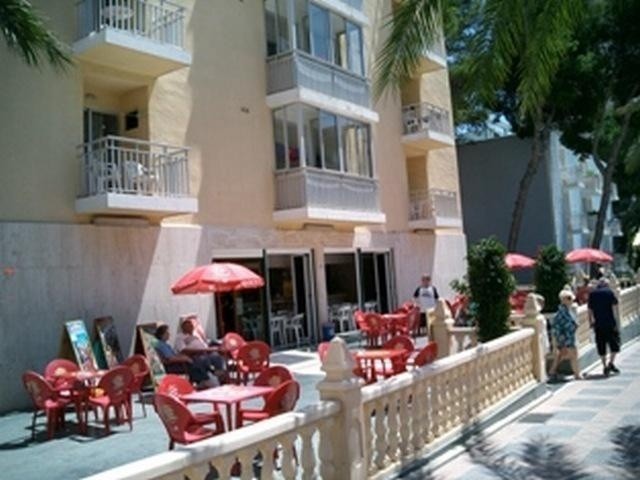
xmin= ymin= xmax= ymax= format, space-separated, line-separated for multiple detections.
xmin=150 ymin=332 xmax=272 ymax=391
xmin=152 ymin=365 xmax=302 ymax=467
xmin=318 ymin=301 xmax=469 ymax=417
xmin=100 ymin=161 xmax=156 ymax=195
xmin=578 ymin=284 xmax=596 ymax=305
xmin=401 ymin=108 xmax=419 ymax=132
xmin=23 ymin=354 xmax=150 ymax=440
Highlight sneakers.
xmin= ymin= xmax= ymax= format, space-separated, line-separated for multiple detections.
xmin=608 ymin=362 xmax=619 ymax=373
xmin=604 ymin=367 xmax=609 ymax=376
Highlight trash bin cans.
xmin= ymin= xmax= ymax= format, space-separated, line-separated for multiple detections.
xmin=322 ymin=322 xmax=335 ymax=342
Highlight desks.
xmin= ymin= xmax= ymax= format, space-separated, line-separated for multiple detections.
xmin=104 ymin=5 xmax=133 ymax=18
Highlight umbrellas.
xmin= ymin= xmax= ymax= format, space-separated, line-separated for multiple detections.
xmin=563 ymin=246 xmax=613 ymax=275
xmin=171 ymin=262 xmax=265 ymax=359
xmin=504 ymin=253 xmax=536 ymax=282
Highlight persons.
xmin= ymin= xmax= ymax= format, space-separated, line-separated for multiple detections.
xmin=547 ymin=268 xmax=621 ymax=384
xmin=412 ymin=274 xmax=439 ymax=337
xmin=450 ymin=293 xmax=480 ymax=327
xmin=153 ymin=317 xmax=226 ymax=389
